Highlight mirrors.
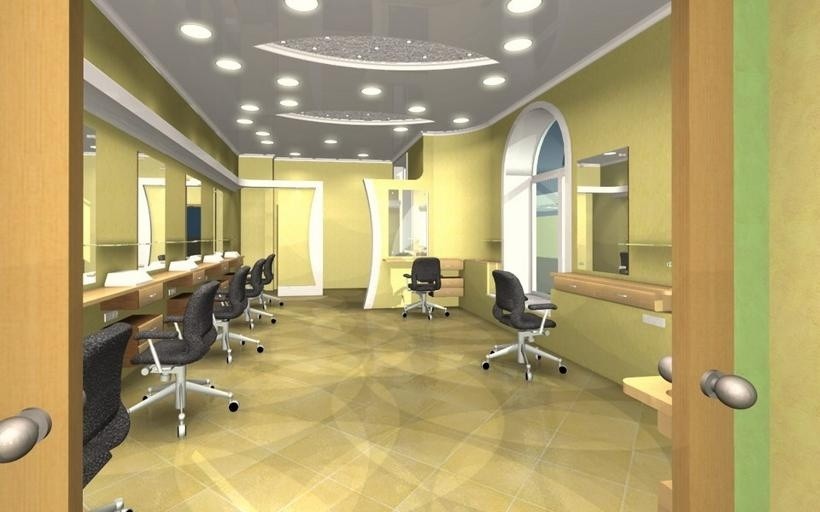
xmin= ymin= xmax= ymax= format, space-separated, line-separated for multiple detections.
xmin=575 ymin=146 xmax=630 ymax=276
xmin=83 ymin=123 xmax=225 ymax=284
xmin=388 ymin=188 xmax=430 ymax=257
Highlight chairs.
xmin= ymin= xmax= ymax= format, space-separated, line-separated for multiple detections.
xmin=82 ymin=254 xmax=286 ymax=511
xmin=480 ymin=270 xmax=567 ymax=381
xmin=401 ymin=258 xmax=449 ymax=321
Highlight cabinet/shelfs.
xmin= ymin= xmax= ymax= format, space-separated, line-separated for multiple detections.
xmin=552 ymin=273 xmax=672 ymax=315
